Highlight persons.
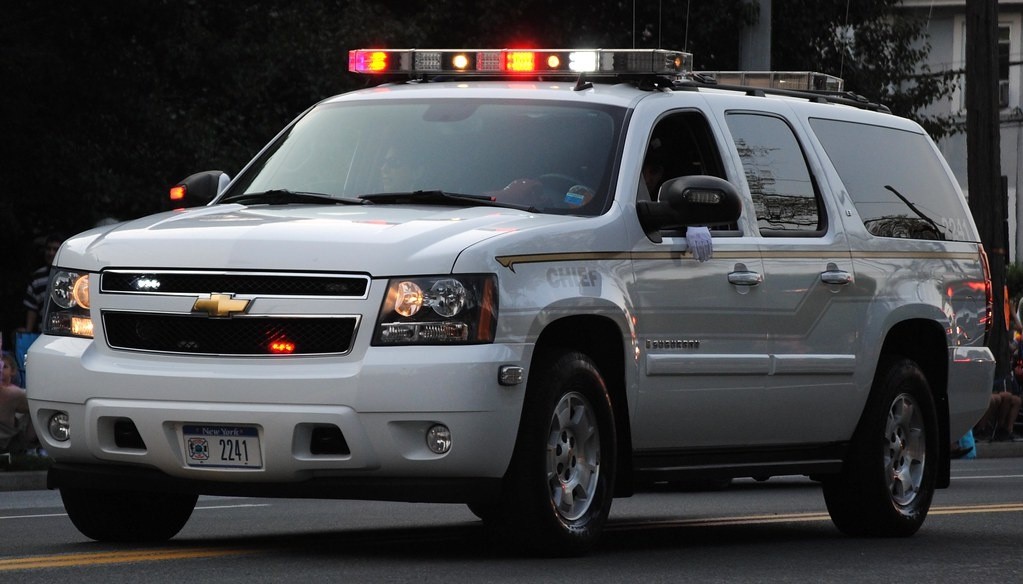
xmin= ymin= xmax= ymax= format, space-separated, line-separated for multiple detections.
xmin=16 ymin=237 xmax=61 ymax=333
xmin=381 ymin=141 xmax=429 ymax=193
xmin=0 ymin=356 xmax=36 ymax=467
xmin=995 ymin=392 xmax=1023 ymax=442
xmin=950 ymin=443 xmax=974 ymax=459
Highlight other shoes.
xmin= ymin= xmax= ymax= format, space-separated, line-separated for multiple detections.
xmin=952 ymin=446 xmax=974 ymax=459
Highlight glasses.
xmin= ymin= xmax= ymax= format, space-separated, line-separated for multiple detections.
xmin=382 ymin=156 xmax=412 ymax=169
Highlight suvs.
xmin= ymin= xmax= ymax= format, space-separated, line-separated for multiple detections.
xmin=22 ymin=47 xmax=996 ymax=557
